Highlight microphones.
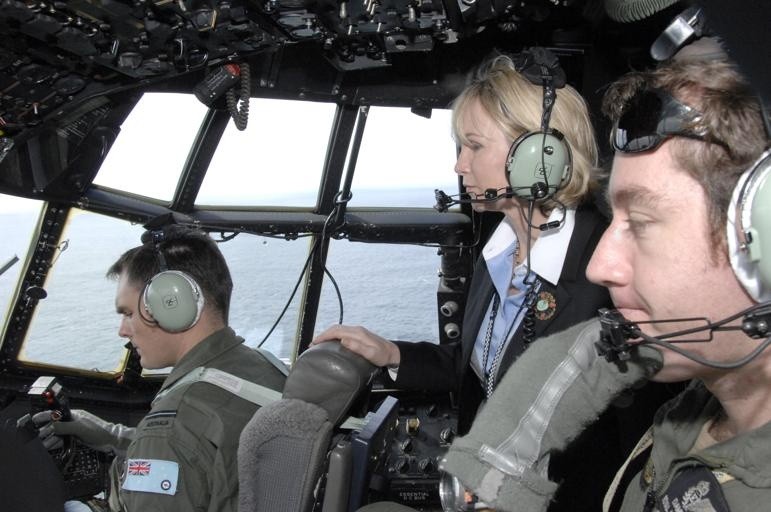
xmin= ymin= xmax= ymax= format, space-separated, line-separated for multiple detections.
xmin=434 ymin=189 xmax=530 ymax=211
xmin=597 ymin=302 xmax=771 ymax=371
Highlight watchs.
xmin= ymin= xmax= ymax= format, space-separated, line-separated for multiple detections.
xmin=434 ymin=471 xmax=485 ymax=511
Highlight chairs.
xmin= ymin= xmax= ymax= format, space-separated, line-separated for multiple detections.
xmin=236 ymin=341 xmax=399 ymax=512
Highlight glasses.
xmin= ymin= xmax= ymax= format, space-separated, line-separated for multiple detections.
xmin=609 ymin=87 xmax=727 ymax=154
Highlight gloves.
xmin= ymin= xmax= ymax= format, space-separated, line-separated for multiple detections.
xmin=32 ymin=410 xmax=137 ymax=457
xmin=438 ymin=318 xmax=662 ymax=511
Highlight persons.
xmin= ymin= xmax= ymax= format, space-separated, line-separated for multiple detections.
xmin=437 ymin=51 xmax=771 ymax=511
xmin=307 ymin=53 xmax=615 ymax=430
xmin=17 ymin=225 xmax=291 ymax=511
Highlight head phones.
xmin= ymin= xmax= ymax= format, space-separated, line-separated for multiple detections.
xmin=143 ymin=228 xmax=203 ymax=333
xmin=508 ymin=51 xmax=573 ymax=205
xmin=727 ymin=149 xmax=770 ymax=304
